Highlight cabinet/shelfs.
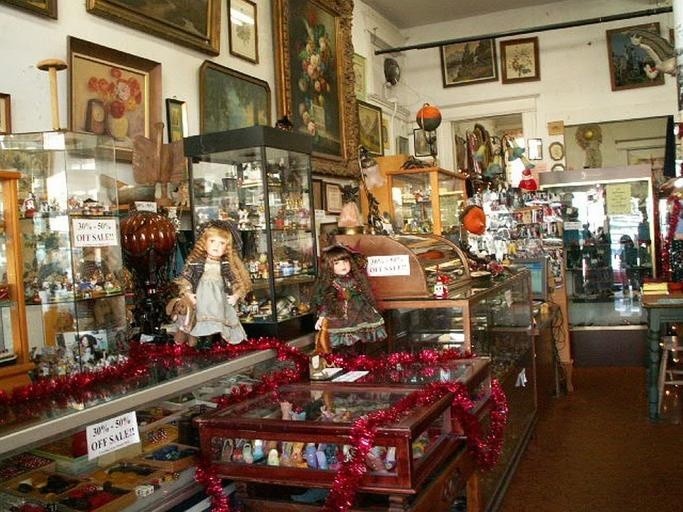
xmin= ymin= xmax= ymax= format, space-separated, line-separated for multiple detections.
xmin=0 ymin=127 xmax=573 ymax=512
xmin=574 ymin=243 xmax=617 ymax=303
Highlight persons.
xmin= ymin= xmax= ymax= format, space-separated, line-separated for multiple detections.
xmin=620 ymin=240 xmax=641 ymax=302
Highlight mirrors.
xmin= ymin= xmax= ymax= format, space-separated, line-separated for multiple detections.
xmin=539 ymin=177 xmax=656 ymax=332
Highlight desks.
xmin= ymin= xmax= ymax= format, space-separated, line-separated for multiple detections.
xmin=638 ymin=285 xmax=683 ymax=420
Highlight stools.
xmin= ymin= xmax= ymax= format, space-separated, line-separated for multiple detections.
xmin=657 ymin=335 xmax=683 ymax=414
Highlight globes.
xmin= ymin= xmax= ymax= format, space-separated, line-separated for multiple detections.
xmin=416 ymin=103 xmax=442 ymax=166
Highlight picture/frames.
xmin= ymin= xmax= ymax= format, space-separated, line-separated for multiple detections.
xmin=605 ymin=23 xmax=665 ymax=91
xmin=500 ymin=37 xmax=541 ymax=84
xmin=166 ymin=98 xmax=189 ymax=145
xmin=67 ymin=35 xmax=163 ymax=141
xmin=0 ymin=0 xmax=58 ymax=20
xmin=0 ymin=92 xmax=13 ymax=135
xmin=85 ymin=0 xmax=222 ymax=55
xmin=356 ymin=97 xmax=385 ymax=157
xmin=200 ymin=60 xmax=272 ymax=136
xmin=228 ymin=0 xmax=260 ymax=63
xmin=270 ymin=0 xmax=361 ymax=180
xmin=439 ymin=37 xmax=498 ymax=88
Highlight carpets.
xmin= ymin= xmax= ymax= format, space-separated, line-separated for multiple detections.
xmin=469 ymin=362 xmax=683 ymax=512
